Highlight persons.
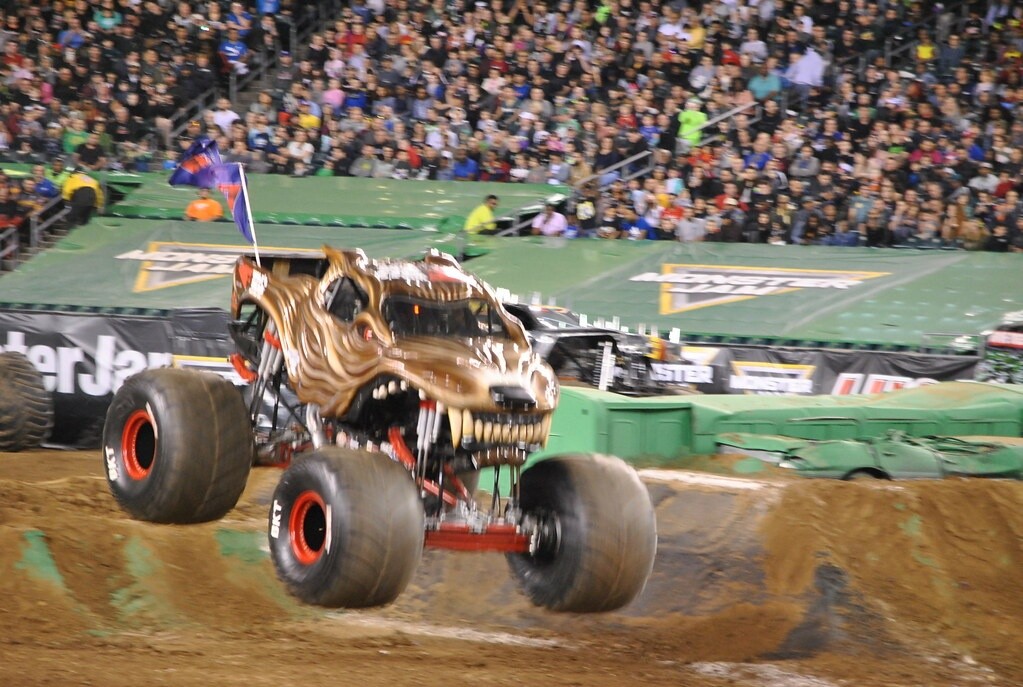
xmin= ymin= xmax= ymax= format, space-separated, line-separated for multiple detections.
xmin=184 ymin=188 xmax=223 ymax=222
xmin=464 ymin=195 xmax=502 ymax=236
xmin=62 ymin=167 xmax=103 ymax=225
xmin=0 ymin=0 xmax=1023 ymax=259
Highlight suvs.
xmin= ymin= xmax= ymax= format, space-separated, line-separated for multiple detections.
xmin=100 ymin=248 xmax=661 ymax=614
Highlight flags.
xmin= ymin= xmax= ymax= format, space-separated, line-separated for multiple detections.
xmin=168 ymin=140 xmax=254 ymax=244
